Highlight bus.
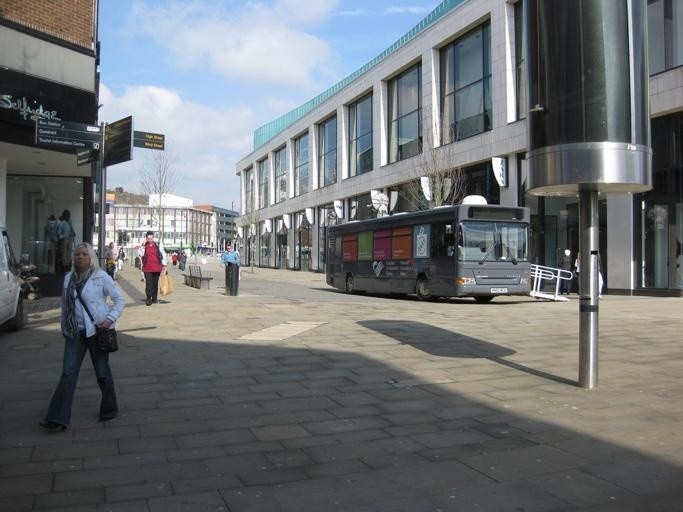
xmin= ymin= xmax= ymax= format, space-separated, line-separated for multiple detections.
xmin=325 ymin=202 xmax=534 ymax=303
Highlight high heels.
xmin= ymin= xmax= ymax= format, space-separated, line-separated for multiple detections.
xmin=100 ymin=413 xmax=115 ymax=419
xmin=40 ymin=421 xmax=67 ymax=431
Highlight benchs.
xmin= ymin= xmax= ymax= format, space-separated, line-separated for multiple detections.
xmin=180 ymin=262 xmax=213 ymax=289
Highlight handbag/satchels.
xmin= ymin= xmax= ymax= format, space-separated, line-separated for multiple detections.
xmin=96 ymin=326 xmax=118 ymax=353
xmin=161 ymin=272 xmax=172 ymax=295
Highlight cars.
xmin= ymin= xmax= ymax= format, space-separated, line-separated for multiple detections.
xmin=0 ymin=227 xmax=29 ymax=332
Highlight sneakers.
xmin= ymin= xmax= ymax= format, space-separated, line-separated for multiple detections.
xmin=152 ymin=294 xmax=157 ymax=302
xmin=146 ymin=300 xmax=152 ymax=305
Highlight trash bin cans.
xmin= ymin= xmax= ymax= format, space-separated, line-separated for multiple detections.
xmin=225 ymin=261 xmax=239 ymax=296
xmin=135 ymin=257 xmax=141 ymax=268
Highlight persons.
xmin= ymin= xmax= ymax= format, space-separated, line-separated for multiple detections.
xmin=219 ymin=244 xmax=239 ymax=295
xmin=43 ymin=215 xmax=58 ymax=248
xmin=104 ymin=241 xmax=186 ymax=282
xmin=574 ymin=252 xmax=579 ymax=273
xmin=19 ymin=253 xmax=40 ymax=301
xmin=137 ymin=231 xmax=167 ymax=306
xmin=557 ymin=248 xmax=572 ymax=296
xmin=36 ymin=241 xmax=125 ymax=431
xmin=56 ymin=209 xmax=75 ymax=275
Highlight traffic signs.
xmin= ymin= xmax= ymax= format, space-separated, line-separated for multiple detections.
xmin=34 ymin=114 xmax=166 ymax=272
